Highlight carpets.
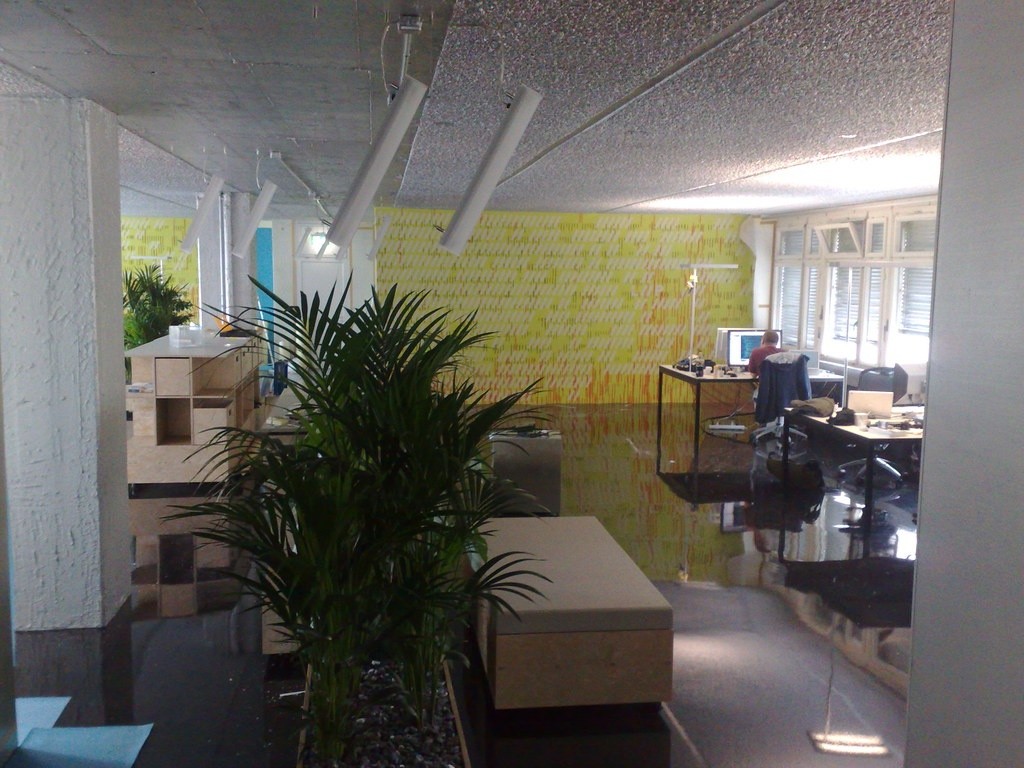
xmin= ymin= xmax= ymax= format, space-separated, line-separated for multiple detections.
xmin=3 ymin=721 xmax=154 ymax=768
xmin=15 ymin=696 xmax=72 ymax=747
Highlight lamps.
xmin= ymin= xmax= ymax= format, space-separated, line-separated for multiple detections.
xmin=367 ymin=215 xmax=392 ymax=260
xmin=327 ymin=75 xmax=429 ymax=246
xmin=232 ymin=179 xmax=278 ymax=260
xmin=437 ymin=84 xmax=544 ymax=255
xmin=180 ymin=176 xmax=224 ymax=254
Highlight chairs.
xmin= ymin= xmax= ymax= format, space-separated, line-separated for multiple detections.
xmin=751 ymin=352 xmax=809 ymax=445
xmin=838 ymin=367 xmax=908 ymax=485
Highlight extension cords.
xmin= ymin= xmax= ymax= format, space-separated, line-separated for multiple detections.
xmin=709 ymin=425 xmax=746 ymax=433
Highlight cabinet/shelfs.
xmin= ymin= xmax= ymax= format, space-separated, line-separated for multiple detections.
xmin=124 ymin=330 xmax=265 ymax=483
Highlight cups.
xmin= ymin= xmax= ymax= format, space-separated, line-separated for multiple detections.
xmin=718 ymin=370 xmax=724 ymax=377
xmin=696 ymin=366 xmax=704 ymax=377
xmin=854 ymin=413 xmax=869 ymax=430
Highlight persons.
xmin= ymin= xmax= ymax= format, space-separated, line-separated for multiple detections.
xmin=745 ymin=330 xmax=788 ymax=377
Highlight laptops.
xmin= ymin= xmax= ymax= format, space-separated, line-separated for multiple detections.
xmin=848 ymin=390 xmax=894 ymax=418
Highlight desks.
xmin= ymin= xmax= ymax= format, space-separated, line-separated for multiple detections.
xmin=658 ymin=365 xmax=846 ymax=483
xmin=489 ymin=428 xmax=562 ymax=515
xmin=782 ymin=406 xmax=924 ymax=534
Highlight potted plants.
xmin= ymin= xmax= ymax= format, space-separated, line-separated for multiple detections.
xmin=194 ymin=272 xmax=575 ymax=768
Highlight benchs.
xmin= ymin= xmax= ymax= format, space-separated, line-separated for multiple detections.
xmin=463 ymin=515 xmax=674 ymax=710
xmin=260 ymin=479 xmax=307 ymax=656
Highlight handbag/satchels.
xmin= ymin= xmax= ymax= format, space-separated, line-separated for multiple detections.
xmin=789 ymin=397 xmax=834 ymax=417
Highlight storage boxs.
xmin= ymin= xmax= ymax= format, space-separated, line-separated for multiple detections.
xmin=894 ymin=363 xmax=927 ymax=405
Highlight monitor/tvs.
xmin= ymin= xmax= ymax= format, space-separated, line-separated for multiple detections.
xmin=716 ymin=328 xmax=762 ymax=359
xmin=729 ymin=330 xmax=782 ymax=371
